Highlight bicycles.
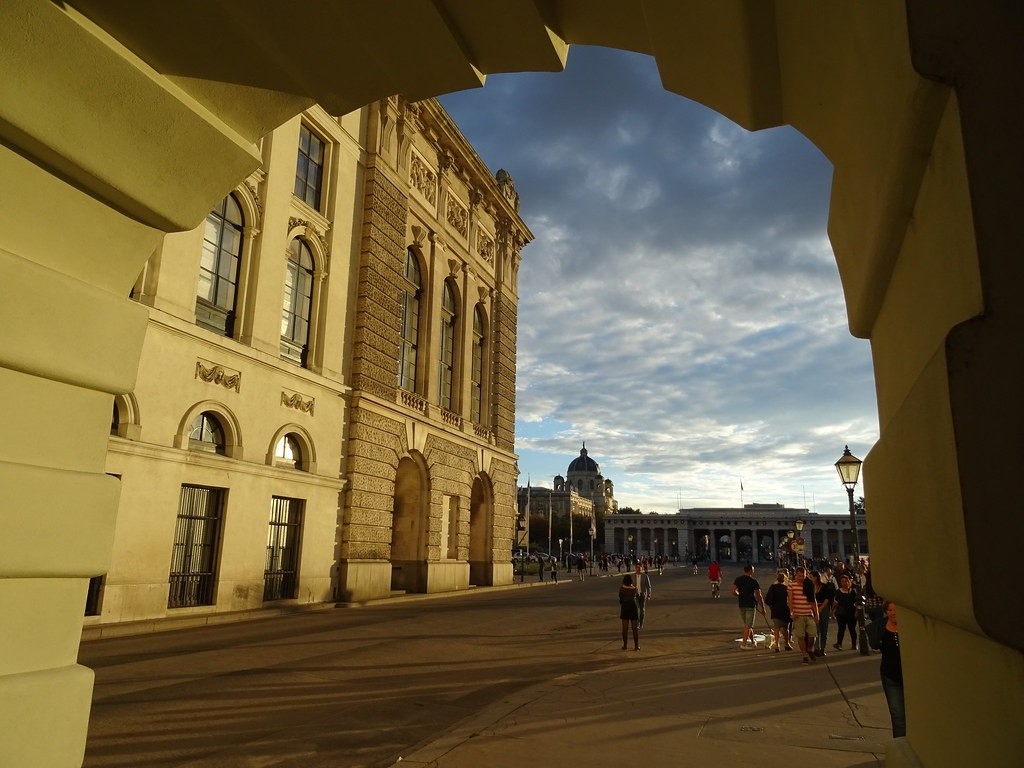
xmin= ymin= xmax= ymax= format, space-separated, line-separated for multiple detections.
xmin=710 ymin=575 xmax=722 ymax=602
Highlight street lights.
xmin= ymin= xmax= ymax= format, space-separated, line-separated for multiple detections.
xmin=654 ymin=538 xmax=657 ymax=568
xmin=628 ymin=535 xmax=633 ymax=560
xmin=672 ymin=541 xmax=676 ymax=566
xmin=834 ymin=445 xmax=868 ymax=656
xmin=796 ymin=518 xmax=804 ymax=567
xmin=779 ymin=530 xmax=794 ymax=566
xmin=588 ymin=528 xmax=594 ymax=576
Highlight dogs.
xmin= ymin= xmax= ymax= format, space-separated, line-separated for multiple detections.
xmin=761 ymin=629 xmax=780 ymax=651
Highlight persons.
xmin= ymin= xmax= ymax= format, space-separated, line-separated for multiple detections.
xmin=864 ymin=601 xmax=906 ymax=738
xmin=707 ymin=561 xmax=723 ymax=587
xmin=764 ymin=554 xmax=884 ymax=665
xmin=550 ymin=559 xmax=558 ymax=585
xmin=619 ymin=574 xmax=641 ymax=651
xmin=631 ymin=563 xmax=652 ymax=630
xmin=732 ymin=562 xmax=767 ymax=650
xmin=531 ymin=553 xmax=535 ymax=563
xmin=691 ymin=556 xmax=698 ymax=575
xmin=563 ymin=550 xmax=670 ymax=582
xmin=538 ymin=556 xmax=545 ymax=581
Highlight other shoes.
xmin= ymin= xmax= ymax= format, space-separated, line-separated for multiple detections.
xmin=812 ymin=649 xmax=820 ymax=655
xmin=806 ymin=648 xmax=817 ymax=661
xmin=816 ymin=651 xmax=825 ymax=657
xmin=775 ymin=646 xmax=780 ymax=652
xmin=852 ymin=644 xmax=856 ymax=649
xmin=752 ymin=640 xmax=757 ymax=648
xmin=784 ymin=643 xmax=793 ymax=651
xmin=802 ymin=657 xmax=809 ymax=664
xmin=834 ymin=643 xmax=842 ymax=651
xmin=741 ymin=645 xmax=751 ymax=650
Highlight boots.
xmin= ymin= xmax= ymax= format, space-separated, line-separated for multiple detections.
xmin=634 ymin=641 xmax=641 ymax=651
xmin=622 ymin=640 xmax=628 ymax=650
